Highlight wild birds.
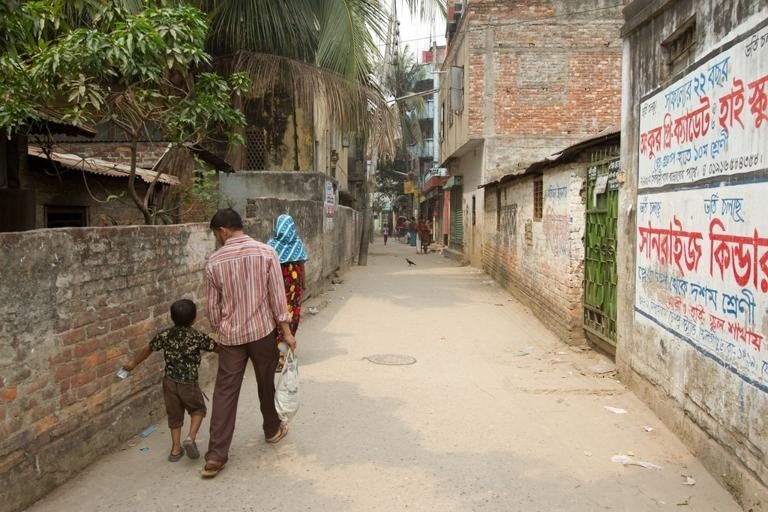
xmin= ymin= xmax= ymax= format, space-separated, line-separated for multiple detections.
xmin=405 ymin=257 xmax=416 ymax=267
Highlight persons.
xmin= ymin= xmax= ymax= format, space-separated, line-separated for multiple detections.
xmin=382 ymin=214 xmax=433 ymax=254
xmin=200 ymin=208 xmax=296 ymax=477
xmin=122 ymin=300 xmax=218 ymax=462
xmin=267 ymin=214 xmax=308 ymax=372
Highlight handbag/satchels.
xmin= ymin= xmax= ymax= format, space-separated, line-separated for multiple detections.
xmin=273 ymin=346 xmax=302 ymax=424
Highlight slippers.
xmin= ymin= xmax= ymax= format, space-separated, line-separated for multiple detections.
xmin=268 ymin=423 xmax=289 ymax=445
xmin=199 ymin=464 xmax=226 ymax=478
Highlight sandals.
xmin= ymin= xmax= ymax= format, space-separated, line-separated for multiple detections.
xmin=166 ymin=446 xmax=186 ymax=463
xmin=182 ymin=440 xmax=199 ymax=460
